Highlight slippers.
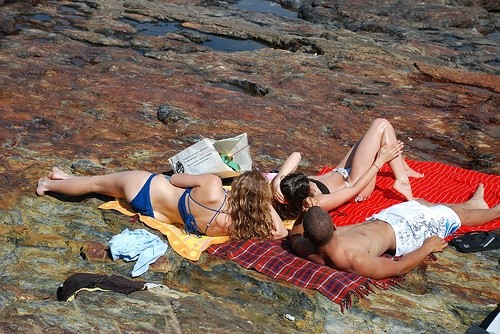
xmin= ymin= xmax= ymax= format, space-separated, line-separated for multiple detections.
xmin=449 ymin=231 xmax=500 ymax=253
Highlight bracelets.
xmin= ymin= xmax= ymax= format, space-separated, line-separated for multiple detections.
xmin=372 ymin=163 xmax=381 ymax=171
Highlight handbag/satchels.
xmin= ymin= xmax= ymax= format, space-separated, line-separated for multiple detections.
xmin=168 ymin=131 xmax=254 ymax=179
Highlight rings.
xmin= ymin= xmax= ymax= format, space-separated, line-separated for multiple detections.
xmin=273 ymin=196 xmax=277 ymax=200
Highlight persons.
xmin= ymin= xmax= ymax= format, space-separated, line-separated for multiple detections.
xmin=36 ymin=165 xmax=289 ymax=241
xmin=292 ymin=182 xmax=500 ymax=279
xmin=269 ymin=117 xmax=424 ymax=222
